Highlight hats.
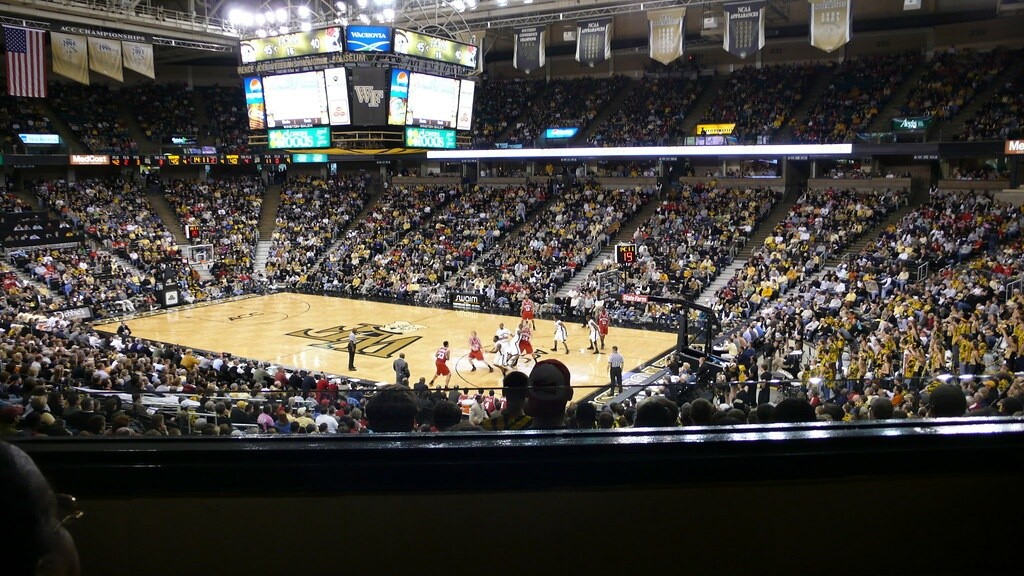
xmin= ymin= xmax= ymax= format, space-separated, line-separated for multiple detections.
xmin=31 ymin=396 xmax=47 ymax=408
xmin=524 ymin=359 xmax=570 ymax=416
xmin=0 ymin=405 xmax=23 ymax=419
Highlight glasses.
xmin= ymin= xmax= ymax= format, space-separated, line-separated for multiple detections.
xmin=43 ymin=491 xmax=85 ymax=537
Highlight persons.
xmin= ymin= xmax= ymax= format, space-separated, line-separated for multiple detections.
xmin=0 ymin=39 xmax=1023 ymax=437
xmin=0 ymin=439 xmax=81 ymax=576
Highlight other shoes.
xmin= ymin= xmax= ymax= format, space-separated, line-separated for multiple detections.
xmin=587 ymin=346 xmax=594 ymax=349
xmin=348 ymin=366 xmax=356 ymax=371
xmin=601 ymin=343 xmax=605 ymax=349
xmin=471 ymin=367 xmax=476 ymax=371
xmin=607 ymin=392 xmax=614 ymax=396
xmin=551 ymin=348 xmax=557 ymax=351
xmin=593 ymin=350 xmax=599 ymax=354
xmin=533 ymin=325 xmax=536 ymax=331
xmin=565 ymin=350 xmax=569 ymax=354
xmin=429 ymin=380 xmax=434 ymax=386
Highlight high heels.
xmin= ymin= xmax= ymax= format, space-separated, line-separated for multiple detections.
xmin=489 ymin=368 xmax=494 ymax=372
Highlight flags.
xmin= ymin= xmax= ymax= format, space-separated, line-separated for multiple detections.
xmin=6 ymin=28 xmax=46 ymax=98
